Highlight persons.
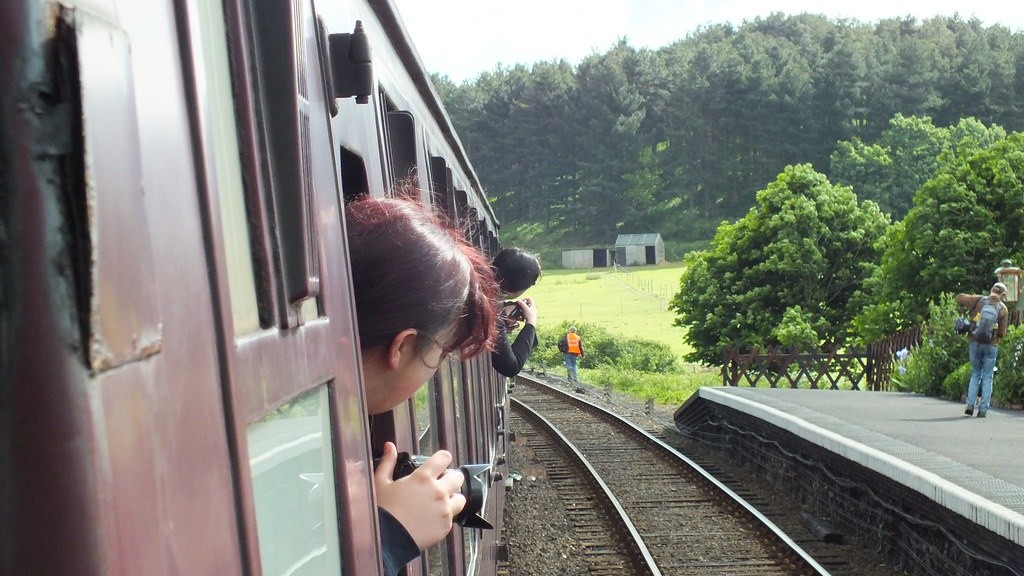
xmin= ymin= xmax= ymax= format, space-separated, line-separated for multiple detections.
xmin=485 ymin=248 xmax=542 ymax=378
xmin=343 ymin=196 xmax=469 ymax=576
xmin=563 ymin=326 xmax=586 ymax=385
xmin=954 ymin=283 xmax=1010 ymax=416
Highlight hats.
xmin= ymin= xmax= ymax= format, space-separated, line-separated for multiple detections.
xmin=994 ymin=282 xmax=1007 ymax=294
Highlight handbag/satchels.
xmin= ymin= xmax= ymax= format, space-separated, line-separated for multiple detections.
xmin=558 ymin=334 xmax=568 ymax=353
xmin=956 ymin=318 xmax=971 ymax=331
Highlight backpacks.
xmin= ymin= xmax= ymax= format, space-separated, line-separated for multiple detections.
xmin=969 ymin=296 xmax=1004 ymax=343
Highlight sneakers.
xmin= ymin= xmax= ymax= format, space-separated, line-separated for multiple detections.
xmin=977 ymin=411 xmax=986 ymax=417
xmin=965 ymin=405 xmax=974 ymax=416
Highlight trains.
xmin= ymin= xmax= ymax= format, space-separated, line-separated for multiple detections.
xmin=4 ymin=0 xmax=522 ymax=576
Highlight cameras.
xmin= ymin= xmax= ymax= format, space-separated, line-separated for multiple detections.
xmin=372 ymin=451 xmax=494 ymax=530
xmin=503 ymin=300 xmax=531 ymax=322
